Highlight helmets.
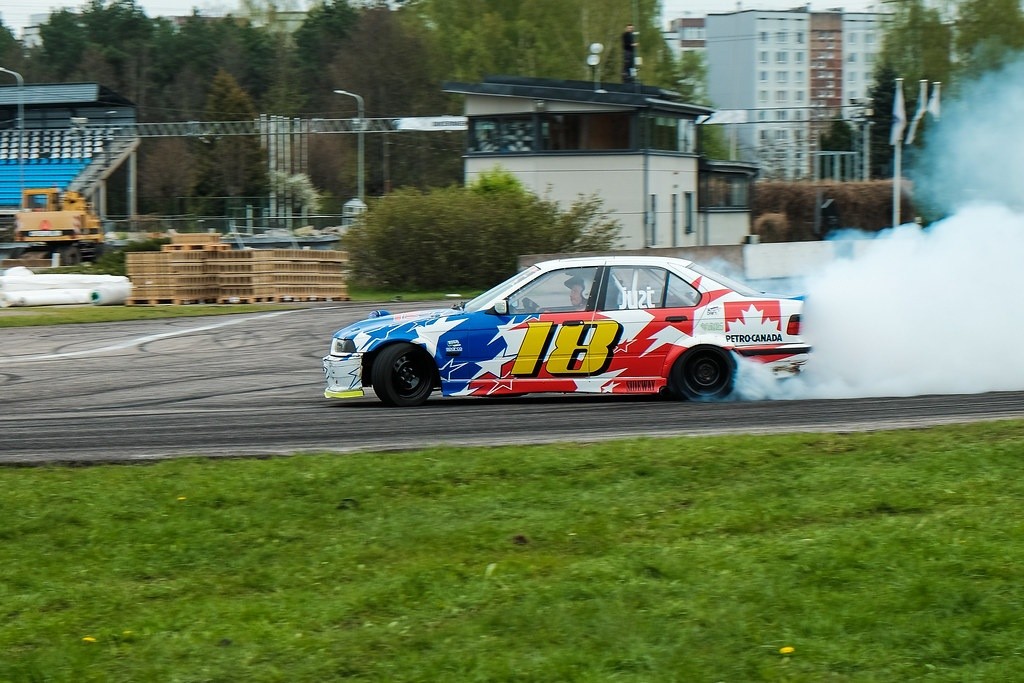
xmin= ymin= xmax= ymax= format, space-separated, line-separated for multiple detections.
xmin=564 ymin=270 xmax=594 ymax=299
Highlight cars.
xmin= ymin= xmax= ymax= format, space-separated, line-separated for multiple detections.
xmin=321 ymin=255 xmax=814 ymax=408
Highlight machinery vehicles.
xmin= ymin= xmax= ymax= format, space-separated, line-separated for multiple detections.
xmin=11 ymin=186 xmax=105 ymax=268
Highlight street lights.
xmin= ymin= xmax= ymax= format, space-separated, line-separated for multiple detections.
xmin=333 ymin=90 xmax=367 ymax=202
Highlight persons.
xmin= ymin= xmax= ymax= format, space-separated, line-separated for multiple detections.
xmin=564 ymin=276 xmax=594 ymax=308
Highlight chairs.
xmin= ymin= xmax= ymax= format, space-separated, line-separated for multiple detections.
xmin=604 ymin=279 xmax=624 ymax=309
xmin=0 ymin=129 xmax=116 ymax=207
